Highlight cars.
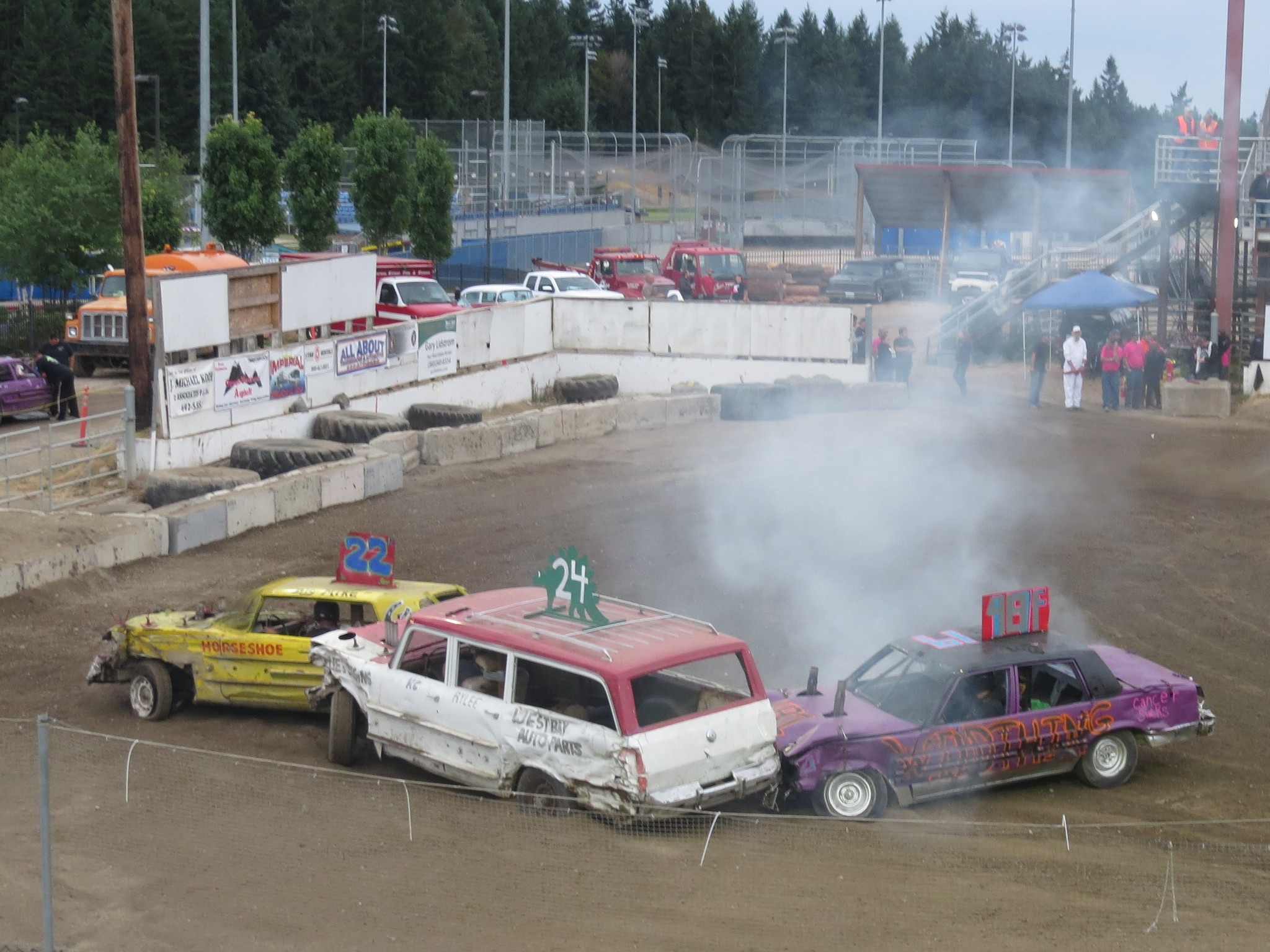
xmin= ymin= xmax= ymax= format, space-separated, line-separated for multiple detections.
xmin=0 ymin=355 xmax=59 ymax=422
xmin=523 ymin=269 xmax=626 ymax=301
xmin=760 ymin=625 xmax=1217 ymax=824
xmin=455 ymin=283 xmax=535 ymax=314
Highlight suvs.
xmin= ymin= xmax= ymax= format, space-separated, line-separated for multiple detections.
xmin=823 ymin=257 xmax=910 ymax=307
xmin=947 ymin=269 xmax=999 ymax=307
xmin=303 ymin=583 xmax=782 ymax=832
xmin=82 ymin=574 xmax=478 ymax=731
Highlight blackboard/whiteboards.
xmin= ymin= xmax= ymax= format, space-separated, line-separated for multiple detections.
xmin=159 ymin=273 xmax=230 ymax=352
xmin=280 ymin=253 xmax=376 ymax=334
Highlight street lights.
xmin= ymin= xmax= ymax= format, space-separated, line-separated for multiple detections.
xmin=1003 ymin=22 xmax=1028 ymax=167
xmin=14 ymin=97 xmax=28 ymax=152
xmin=657 ymin=54 xmax=669 ymax=152
xmin=774 ymin=25 xmax=800 ymax=186
xmin=582 ymin=45 xmax=599 ymax=206
xmin=377 ymin=13 xmax=400 ymax=121
xmin=133 ymin=73 xmax=163 ymax=168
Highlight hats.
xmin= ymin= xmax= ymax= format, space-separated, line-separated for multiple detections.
xmin=1072 ymin=325 xmax=1081 ymax=333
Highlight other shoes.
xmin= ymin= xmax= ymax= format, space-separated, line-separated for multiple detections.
xmin=1067 ymin=406 xmax=1083 ymax=412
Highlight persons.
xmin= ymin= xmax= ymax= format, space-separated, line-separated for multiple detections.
xmin=1062 ymin=325 xmax=1087 ymax=411
xmin=263 ymin=600 xmax=340 ymax=637
xmin=946 ymin=671 xmax=1004 ymax=724
xmin=442 ymin=648 xmax=483 ymax=686
xmin=1100 ymin=328 xmax=1167 ymax=412
xmin=1249 ymin=166 xmax=1270 ymax=232
xmin=850 ymin=314 xmax=915 ymax=382
xmin=952 ymin=330 xmax=971 ymax=397
xmin=32 ymin=333 xmax=79 ymax=421
xmin=1189 ymin=329 xmax=1232 ymax=379
xmin=1171 ymin=106 xmax=1218 ymax=181
xmin=642 ymin=268 xmax=748 ymax=302
xmin=1027 ymin=332 xmax=1051 ymax=409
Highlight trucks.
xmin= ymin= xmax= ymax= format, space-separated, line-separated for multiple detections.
xmin=660 ymin=239 xmax=751 ymax=303
xmin=530 ymin=246 xmax=685 ymax=302
xmin=280 ymin=249 xmax=468 ymax=354
xmin=65 ymin=242 xmax=248 ymax=382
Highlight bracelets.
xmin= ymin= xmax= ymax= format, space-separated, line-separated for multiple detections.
xmin=1110 ymin=358 xmax=1112 ymax=361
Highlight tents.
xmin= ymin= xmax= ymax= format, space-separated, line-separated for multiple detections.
xmin=1023 ymin=271 xmax=1156 ymax=377
